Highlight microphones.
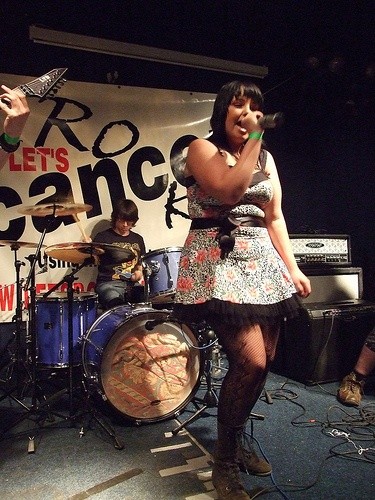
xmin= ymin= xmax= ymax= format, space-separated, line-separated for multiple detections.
xmin=256 ymin=112 xmax=285 ymax=130
xmin=146 ymin=318 xmax=171 ymax=331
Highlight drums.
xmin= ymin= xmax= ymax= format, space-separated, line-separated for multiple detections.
xmin=33 ymin=291 xmax=99 ymax=370
xmin=141 ymin=246 xmax=185 ymax=305
xmin=79 ymin=302 xmax=207 ymax=424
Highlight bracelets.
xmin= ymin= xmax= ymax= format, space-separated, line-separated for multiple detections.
xmin=0 ymin=132 xmax=23 ymax=154
xmin=249 ymin=133 xmax=263 ymax=139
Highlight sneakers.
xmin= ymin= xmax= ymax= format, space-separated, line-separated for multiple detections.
xmin=339 ymin=373 xmax=364 ymax=406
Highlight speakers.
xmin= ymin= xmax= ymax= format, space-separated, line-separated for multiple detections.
xmin=272 ymin=267 xmax=375 ymax=386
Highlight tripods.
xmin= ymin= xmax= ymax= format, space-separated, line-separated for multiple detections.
xmin=0 ymin=206 xmax=125 ymax=451
xmin=172 ymin=331 xmax=264 ymax=434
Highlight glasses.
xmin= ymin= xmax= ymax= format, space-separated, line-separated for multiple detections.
xmin=117 ymin=217 xmax=133 ymax=226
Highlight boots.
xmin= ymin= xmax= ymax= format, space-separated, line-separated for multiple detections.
xmin=207 ymin=424 xmax=251 ymax=499
xmin=239 ymin=432 xmax=272 ymax=476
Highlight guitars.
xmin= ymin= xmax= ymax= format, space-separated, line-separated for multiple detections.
xmin=0 ymin=65 xmax=69 ymax=112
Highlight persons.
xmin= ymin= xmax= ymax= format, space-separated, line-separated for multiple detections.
xmin=336 ymin=327 xmax=375 ymax=406
xmin=172 ymin=79 xmax=311 ymax=500
xmin=93 ymin=200 xmax=146 ymax=311
xmin=0 ymin=86 xmax=31 ymax=172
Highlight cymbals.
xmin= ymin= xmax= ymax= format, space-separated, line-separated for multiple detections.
xmin=0 ymin=240 xmax=47 ymax=249
xmin=44 ymin=242 xmax=137 ymax=266
xmin=16 ymin=203 xmax=93 ymax=216
